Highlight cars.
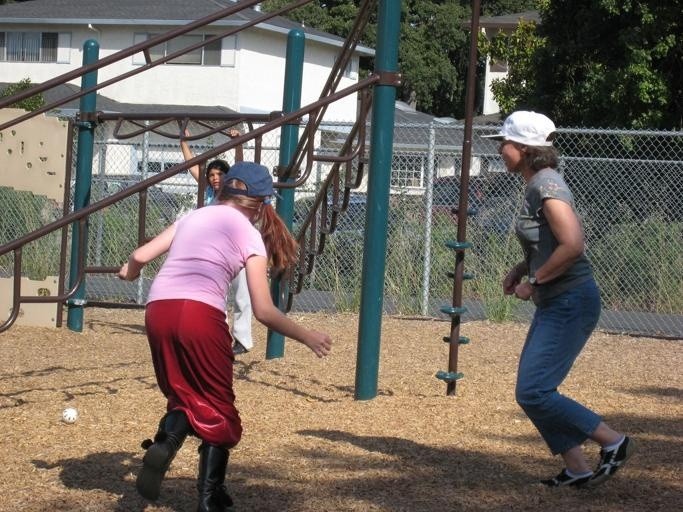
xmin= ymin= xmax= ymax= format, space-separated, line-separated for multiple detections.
xmin=290 ymin=194 xmax=403 ymax=272
xmin=70 ymin=175 xmax=182 ymax=228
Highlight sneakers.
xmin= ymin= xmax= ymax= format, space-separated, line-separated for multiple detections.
xmin=539 ymin=468 xmax=594 ymax=490
xmin=588 ymin=435 xmax=637 ymax=485
xmin=231 ymin=338 xmax=247 ymax=354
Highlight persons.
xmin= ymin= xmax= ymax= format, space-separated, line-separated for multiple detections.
xmin=477 ymin=109 xmax=640 ymax=492
xmin=116 ymin=158 xmax=336 ymax=512
xmin=177 ymin=126 xmax=258 ymax=356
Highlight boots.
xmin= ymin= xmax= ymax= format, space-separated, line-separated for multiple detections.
xmin=198 ymin=445 xmax=233 ymax=512
xmin=136 ymin=410 xmax=189 ymax=501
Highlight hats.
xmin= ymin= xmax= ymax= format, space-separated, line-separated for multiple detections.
xmin=224 ymin=162 xmax=275 ymax=198
xmin=479 ymin=111 xmax=559 ymax=147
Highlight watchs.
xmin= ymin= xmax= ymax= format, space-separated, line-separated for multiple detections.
xmin=529 ymin=275 xmax=540 ymax=289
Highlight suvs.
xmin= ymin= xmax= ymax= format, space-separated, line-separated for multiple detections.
xmin=411 ymin=172 xmax=519 ymax=229
